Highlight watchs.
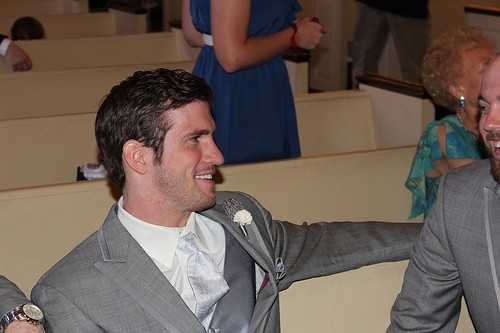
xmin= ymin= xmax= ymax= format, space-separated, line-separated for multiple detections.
xmin=0 ymin=302 xmax=44 ymax=329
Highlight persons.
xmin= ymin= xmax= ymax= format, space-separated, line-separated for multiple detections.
xmin=31 ymin=69 xmax=425 ymax=333
xmin=0 ymin=275 xmax=47 ymax=333
xmin=182 ymin=1 xmax=327 ymax=165
xmin=349 ymin=0 xmax=432 ymax=89
xmin=386 ymin=53 xmax=500 ymax=333
xmin=404 ymin=27 xmax=496 ymax=220
xmin=0 ymin=33 xmax=32 ymax=72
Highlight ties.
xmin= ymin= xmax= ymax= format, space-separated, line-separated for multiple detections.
xmin=177 ymin=230 xmax=231 ymax=333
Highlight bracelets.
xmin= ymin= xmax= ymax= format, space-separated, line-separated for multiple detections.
xmin=291 ymin=21 xmax=302 ymax=52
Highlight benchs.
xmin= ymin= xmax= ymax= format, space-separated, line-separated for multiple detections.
xmin=0 ymin=0 xmax=478 ymax=333
